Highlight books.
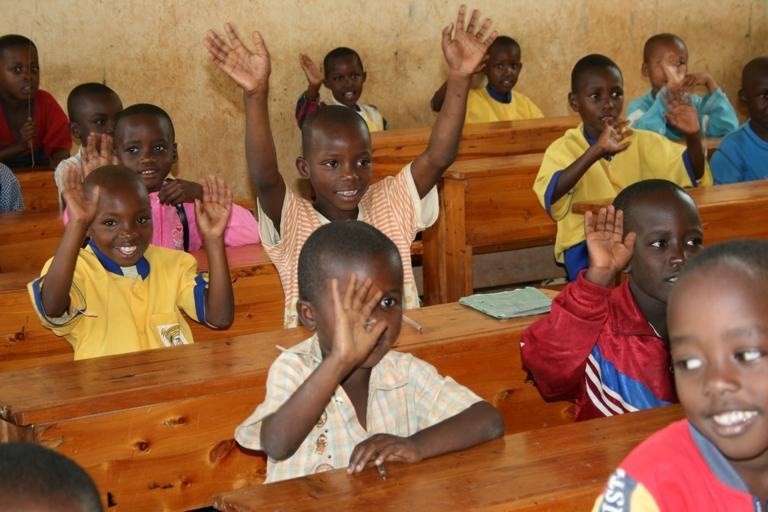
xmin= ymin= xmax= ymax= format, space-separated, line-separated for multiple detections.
xmin=460 ymin=287 xmax=553 ymax=318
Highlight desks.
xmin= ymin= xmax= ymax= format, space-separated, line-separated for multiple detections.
xmin=0 ymin=243 xmax=284 ymax=365
xmin=1 ymin=287 xmax=580 ymax=512
xmin=0 ymin=197 xmax=257 ymax=271
xmin=367 ymin=114 xmax=579 ymax=268
xmin=12 ymin=168 xmax=60 ymax=210
xmin=424 ymin=138 xmax=725 ymax=306
xmin=212 ymin=403 xmax=714 ymax=511
xmin=570 ymin=180 xmax=768 ymax=248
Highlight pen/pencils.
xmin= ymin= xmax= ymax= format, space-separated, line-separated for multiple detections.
xmin=373 ymin=451 xmax=387 ymax=481
xmin=402 ymin=315 xmax=423 ymax=332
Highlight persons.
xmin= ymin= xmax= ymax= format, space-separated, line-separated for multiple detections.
xmin=519 ymin=177 xmax=706 ymax=421
xmin=0 ymin=163 xmax=24 ymax=215
xmin=0 ymin=437 xmax=103 ymax=512
xmin=27 ymin=165 xmax=234 ymax=361
xmin=592 ymin=237 xmax=768 ymax=512
xmin=292 ymin=45 xmax=391 ymax=135
xmin=0 ymin=34 xmax=71 ymax=169
xmin=236 ymin=219 xmax=503 ymax=479
xmin=56 ymin=83 xmax=124 ymax=214
xmin=623 ymin=34 xmax=739 ymax=142
xmin=429 ymin=36 xmax=547 ymax=124
xmin=532 ymin=54 xmax=714 ymax=284
xmin=213 ymin=5 xmax=502 ymax=327
xmin=63 ymin=104 xmax=263 ymax=253
xmin=711 ymin=53 xmax=768 ymax=184
